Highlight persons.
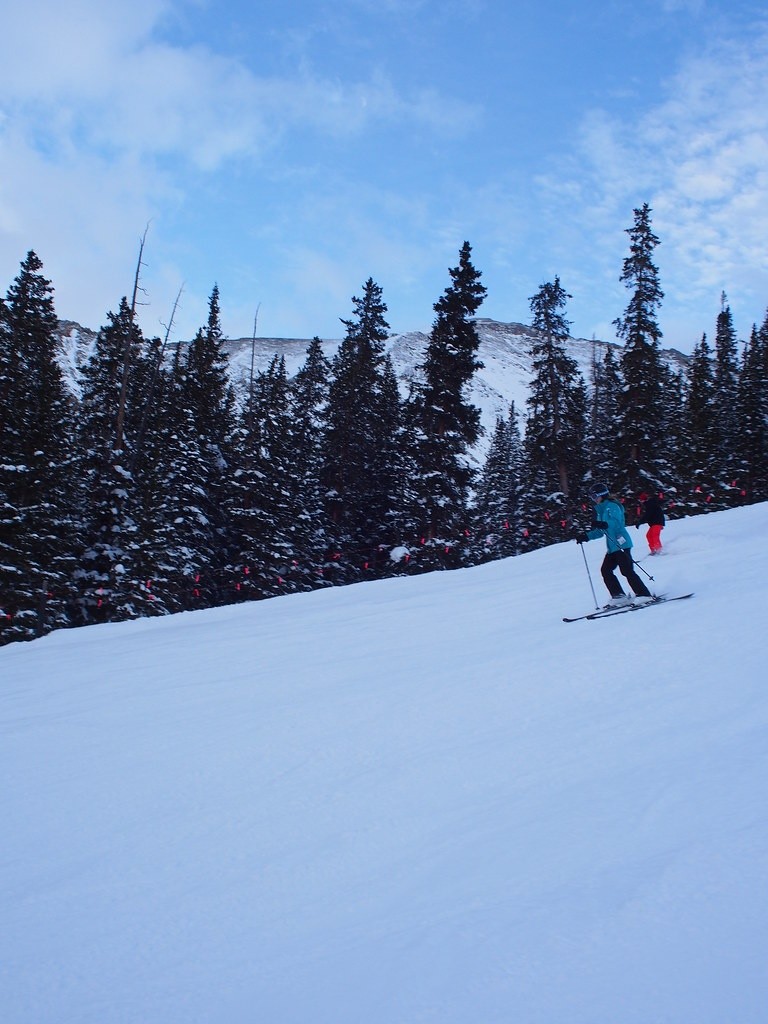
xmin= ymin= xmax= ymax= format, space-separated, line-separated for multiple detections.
xmin=576 ymin=483 xmax=655 ymax=606
xmin=635 ymin=490 xmax=670 ymax=556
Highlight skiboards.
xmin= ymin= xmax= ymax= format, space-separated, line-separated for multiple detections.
xmin=562 ymin=591 xmax=695 ymax=623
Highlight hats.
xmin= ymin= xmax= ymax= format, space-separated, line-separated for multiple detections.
xmin=589 ymin=483 xmax=607 ymax=494
xmin=639 ymin=494 xmax=648 ymax=501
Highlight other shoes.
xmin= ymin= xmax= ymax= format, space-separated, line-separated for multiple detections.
xmin=649 ymin=552 xmax=656 ymax=556
xmin=632 ymin=596 xmax=653 ymax=606
xmin=612 ymin=595 xmax=627 ymax=605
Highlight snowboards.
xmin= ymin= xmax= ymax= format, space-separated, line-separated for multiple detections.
xmin=648 ymin=551 xmax=670 ymax=556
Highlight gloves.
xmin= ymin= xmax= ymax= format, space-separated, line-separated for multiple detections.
xmin=635 ymin=521 xmax=640 ymax=529
xmin=575 ymin=535 xmax=589 ymax=544
xmin=590 ymin=521 xmax=608 ymax=531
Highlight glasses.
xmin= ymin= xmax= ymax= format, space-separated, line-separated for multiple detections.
xmin=591 ymin=493 xmax=598 ymax=501
